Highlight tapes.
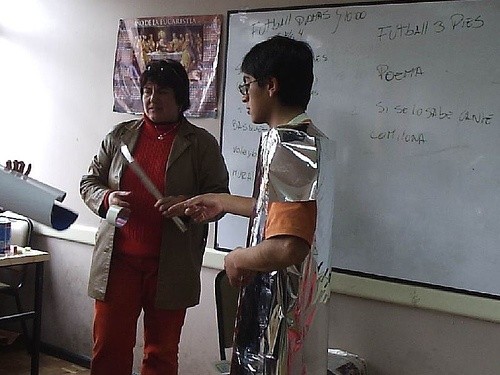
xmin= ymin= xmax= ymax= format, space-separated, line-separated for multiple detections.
xmin=105 ymin=204 xmax=129 ymax=228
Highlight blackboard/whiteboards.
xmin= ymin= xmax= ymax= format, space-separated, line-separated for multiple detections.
xmin=204 ymin=1 xmax=500 ymax=325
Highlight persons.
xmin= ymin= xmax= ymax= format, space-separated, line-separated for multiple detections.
xmin=78 ymin=57 xmax=230 ymax=375
xmin=183 ymin=35 xmax=330 ymax=374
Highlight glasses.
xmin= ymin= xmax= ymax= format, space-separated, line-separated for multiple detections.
xmin=238 ymin=79 xmax=270 ymax=94
xmin=146 ymin=58 xmax=175 ymax=74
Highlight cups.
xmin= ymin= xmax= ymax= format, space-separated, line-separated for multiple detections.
xmin=0 ymin=221 xmax=11 ymax=254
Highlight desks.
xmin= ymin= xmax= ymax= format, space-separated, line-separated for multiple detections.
xmin=0 ymin=248 xmax=50 ymax=375
